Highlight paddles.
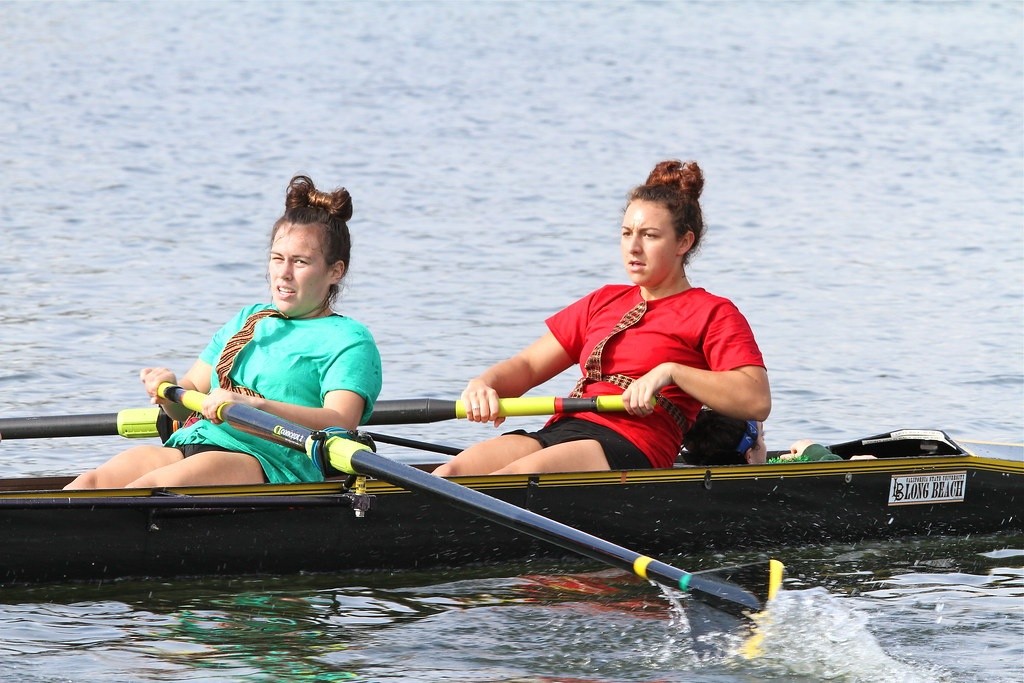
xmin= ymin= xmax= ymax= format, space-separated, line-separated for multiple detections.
xmin=158 ymin=381 xmax=785 ymax=658
xmin=0 ymin=394 xmax=657 ymax=440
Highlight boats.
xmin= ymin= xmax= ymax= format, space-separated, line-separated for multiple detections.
xmin=1 ymin=427 xmax=1024 ymax=580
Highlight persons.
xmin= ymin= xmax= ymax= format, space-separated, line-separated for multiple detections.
xmin=431 ymin=162 xmax=771 ymax=476
xmin=63 ymin=176 xmax=383 ymax=489
xmin=681 ymin=410 xmax=842 ymax=465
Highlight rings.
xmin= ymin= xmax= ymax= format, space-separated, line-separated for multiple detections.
xmin=472 ymin=407 xmax=479 ymax=410
